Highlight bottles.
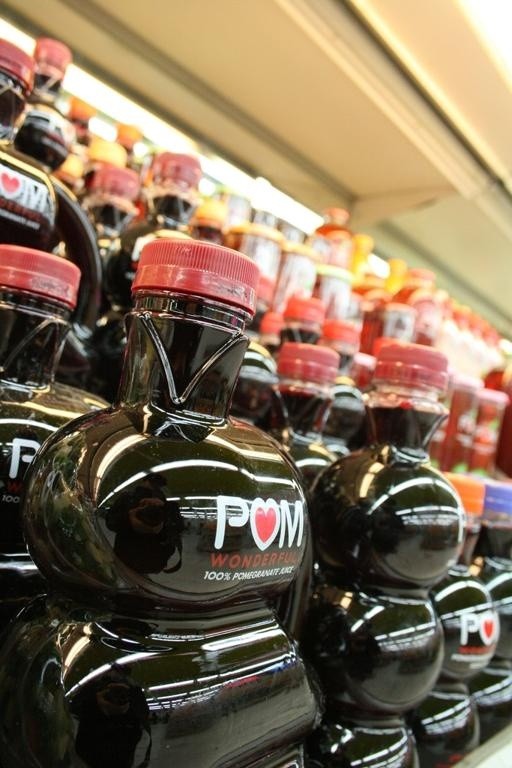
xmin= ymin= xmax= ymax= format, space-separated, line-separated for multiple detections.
xmin=0 ymin=246 xmax=120 ymax=628
xmin=282 ymin=338 xmax=466 ymax=768
xmin=413 ymin=379 xmax=499 ymax=768
xmin=477 ymin=388 xmax=512 ymax=739
xmin=0 ymin=41 xmax=55 ymax=253
xmin=80 ymin=127 xmax=512 ymax=391
xmin=1 ymin=239 xmax=330 ymax=768
xmin=30 ymin=35 xmax=102 ymax=378
